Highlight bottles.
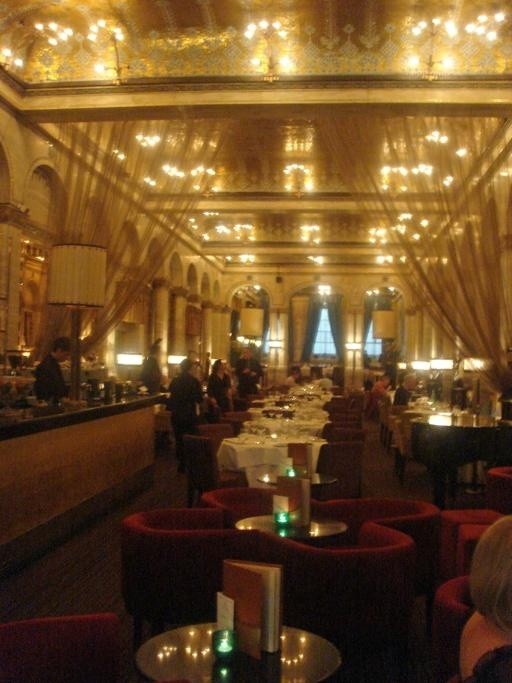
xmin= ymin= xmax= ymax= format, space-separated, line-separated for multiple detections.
xmin=104 ymin=379 xmax=113 ymax=404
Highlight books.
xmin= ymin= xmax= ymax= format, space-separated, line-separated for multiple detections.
xmin=275 ymin=475 xmax=314 ymax=529
xmin=221 ymin=557 xmax=285 ymax=660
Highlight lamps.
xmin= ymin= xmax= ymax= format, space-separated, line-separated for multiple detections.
xmin=48 ymin=242 xmax=108 ymax=396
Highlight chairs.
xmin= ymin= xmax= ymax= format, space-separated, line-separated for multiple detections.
xmin=178 ymin=369 xmax=512 ymax=494
xmin=123 ymin=486 xmax=441 ymax=621
xmin=1 ymin=609 xmax=125 ymax=682
xmin=434 ymin=507 xmax=509 ymax=635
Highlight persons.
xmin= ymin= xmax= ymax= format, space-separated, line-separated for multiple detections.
xmin=137 ymin=343 xmax=415 ymax=477
xmin=33 ymin=335 xmax=73 ymax=399
xmin=458 ymin=516 xmax=512 ymax=683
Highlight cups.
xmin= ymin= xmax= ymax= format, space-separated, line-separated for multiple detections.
xmin=115 ymin=382 xmax=124 ymax=401
xmin=275 ymin=525 xmax=288 ymax=537
xmin=286 ymin=466 xmax=297 ymax=477
xmin=211 ymin=625 xmax=237 ymax=660
xmin=211 ymin=662 xmax=235 ymax=681
xmin=272 ymin=506 xmax=289 ymax=525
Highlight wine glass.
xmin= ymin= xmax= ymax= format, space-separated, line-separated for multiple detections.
xmin=239 ymin=380 xmax=318 ymax=447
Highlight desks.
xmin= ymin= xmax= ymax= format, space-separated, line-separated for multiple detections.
xmin=135 ymin=622 xmax=345 ymax=681
